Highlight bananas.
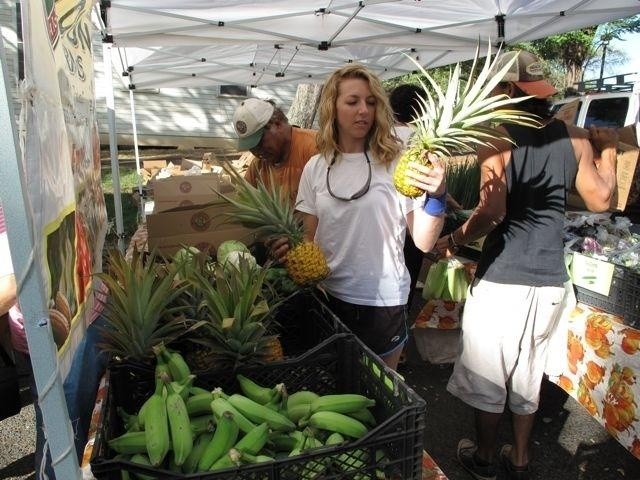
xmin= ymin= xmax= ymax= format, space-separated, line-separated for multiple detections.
xmin=105 ymin=342 xmax=388 ymax=480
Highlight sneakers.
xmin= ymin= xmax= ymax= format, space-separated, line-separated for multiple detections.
xmin=457 ymin=438 xmax=498 ymax=480
xmin=499 ymin=443 xmax=530 ymax=479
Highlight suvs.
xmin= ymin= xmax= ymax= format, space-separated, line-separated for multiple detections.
xmin=541 ymin=74 xmax=639 ymax=140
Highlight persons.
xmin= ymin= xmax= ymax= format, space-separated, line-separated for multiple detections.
xmin=431 ymin=47 xmax=618 ymax=480
xmin=262 ymin=62 xmax=445 ymax=373
xmin=388 ymin=84 xmax=427 ymax=314
xmin=232 ymin=96 xmax=326 ymax=218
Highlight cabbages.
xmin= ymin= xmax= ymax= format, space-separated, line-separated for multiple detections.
xmin=173 ymin=239 xmax=257 ymax=276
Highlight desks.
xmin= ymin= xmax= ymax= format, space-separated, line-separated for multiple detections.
xmin=410 ymin=252 xmax=640 ymax=464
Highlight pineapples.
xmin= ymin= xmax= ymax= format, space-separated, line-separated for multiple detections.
xmin=211 ymin=162 xmax=331 ymax=288
xmin=394 ymin=40 xmax=547 ymax=197
xmin=86 ymin=241 xmax=283 ymax=371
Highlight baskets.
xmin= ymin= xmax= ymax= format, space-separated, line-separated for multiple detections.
xmin=107 ymin=287 xmax=352 ymax=364
xmin=89 ymin=333 xmax=427 ymax=479
xmin=622 ymin=267 xmax=640 ymax=330
xmin=569 ymin=223 xmax=636 ymax=317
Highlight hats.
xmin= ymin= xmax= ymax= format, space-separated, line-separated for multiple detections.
xmin=486 ymin=50 xmax=559 ymax=99
xmin=232 ymin=98 xmax=275 ymax=153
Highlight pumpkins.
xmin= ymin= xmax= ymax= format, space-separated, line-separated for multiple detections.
xmin=559 ymin=317 xmax=640 ymax=459
xmin=414 ymin=301 xmax=457 ymax=331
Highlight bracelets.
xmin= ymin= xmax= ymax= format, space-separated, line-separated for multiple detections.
xmin=423 ymin=190 xmax=447 ymax=217
xmin=447 ymin=233 xmax=461 ymax=251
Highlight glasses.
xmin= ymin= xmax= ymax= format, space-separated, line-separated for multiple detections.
xmin=326 ymin=163 xmax=372 ymax=201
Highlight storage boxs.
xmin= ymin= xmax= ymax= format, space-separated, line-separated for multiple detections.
xmin=552 ymin=98 xmax=639 ymax=214
xmin=145 ymin=203 xmax=251 ymax=238
xmin=147 ymin=227 xmax=258 ymax=262
xmin=142 ymin=160 xmax=166 ymax=170
xmin=234 ymin=150 xmax=256 ymax=168
xmin=148 ymin=167 xmax=219 ymax=213
